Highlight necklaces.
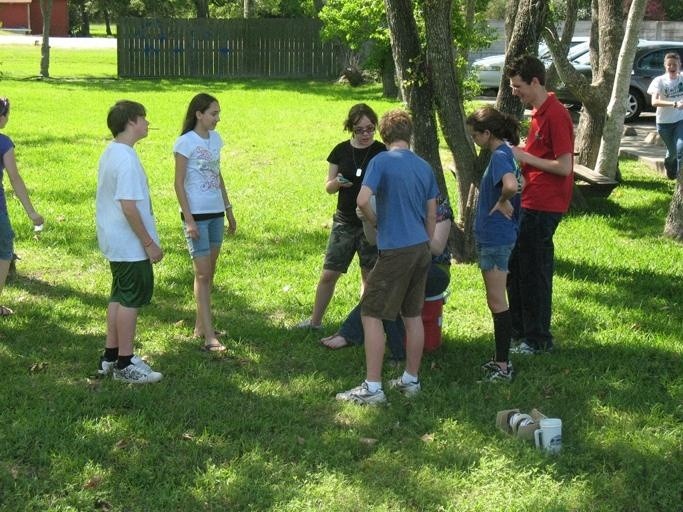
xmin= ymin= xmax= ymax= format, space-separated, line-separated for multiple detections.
xmin=351 ymin=142 xmax=372 ymax=177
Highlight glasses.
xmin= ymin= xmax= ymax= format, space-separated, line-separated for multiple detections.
xmin=353 ymin=127 xmax=375 ymax=134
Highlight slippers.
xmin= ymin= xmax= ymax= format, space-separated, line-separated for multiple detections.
xmin=320 ymin=332 xmax=352 ymax=348
xmin=191 ymin=331 xmax=226 ymax=340
xmin=201 ymin=341 xmax=228 ymax=355
xmin=0 ymin=306 xmax=13 ymax=316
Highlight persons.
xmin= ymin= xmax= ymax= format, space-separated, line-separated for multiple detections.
xmin=336 ymin=109 xmax=436 ymax=406
xmin=506 ymin=54 xmax=573 ymax=356
xmin=172 ymin=93 xmax=236 ymax=357
xmin=0 ymin=97 xmax=44 ymax=320
xmin=93 ymin=101 xmax=164 ymax=387
xmin=305 ymin=105 xmax=386 ymax=333
xmin=319 ymin=183 xmax=452 ymax=361
xmin=466 ymin=109 xmax=520 ymax=386
xmin=647 ymin=52 xmax=683 ymax=182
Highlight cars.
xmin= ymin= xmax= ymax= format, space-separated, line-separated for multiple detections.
xmin=472 ymin=35 xmax=682 ymax=123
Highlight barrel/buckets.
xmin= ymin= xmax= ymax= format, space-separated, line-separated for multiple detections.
xmin=423 ymin=291 xmax=451 ymax=350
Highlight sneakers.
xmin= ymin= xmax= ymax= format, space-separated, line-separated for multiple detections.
xmin=509 ymin=342 xmax=553 ymax=354
xmin=113 ymin=356 xmax=162 ymax=383
xmin=336 ymin=382 xmax=386 ymax=405
xmin=476 ymin=355 xmax=514 ymax=386
xmin=286 ymin=320 xmax=323 ymax=331
xmin=98 ymin=356 xmax=118 ymax=376
xmin=387 ymin=377 xmax=422 ymax=397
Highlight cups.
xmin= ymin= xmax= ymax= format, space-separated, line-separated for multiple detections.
xmin=534 ymin=417 xmax=561 ymax=456
xmin=509 ymin=412 xmax=533 ymax=432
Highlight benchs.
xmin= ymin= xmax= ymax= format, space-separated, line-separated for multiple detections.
xmin=573 ymin=161 xmax=619 ymax=199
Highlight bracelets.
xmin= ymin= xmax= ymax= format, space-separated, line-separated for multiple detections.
xmin=673 ymin=100 xmax=677 ymax=108
xmin=145 ymin=239 xmax=154 ymax=248
xmin=225 ymin=205 xmax=232 ymax=210
